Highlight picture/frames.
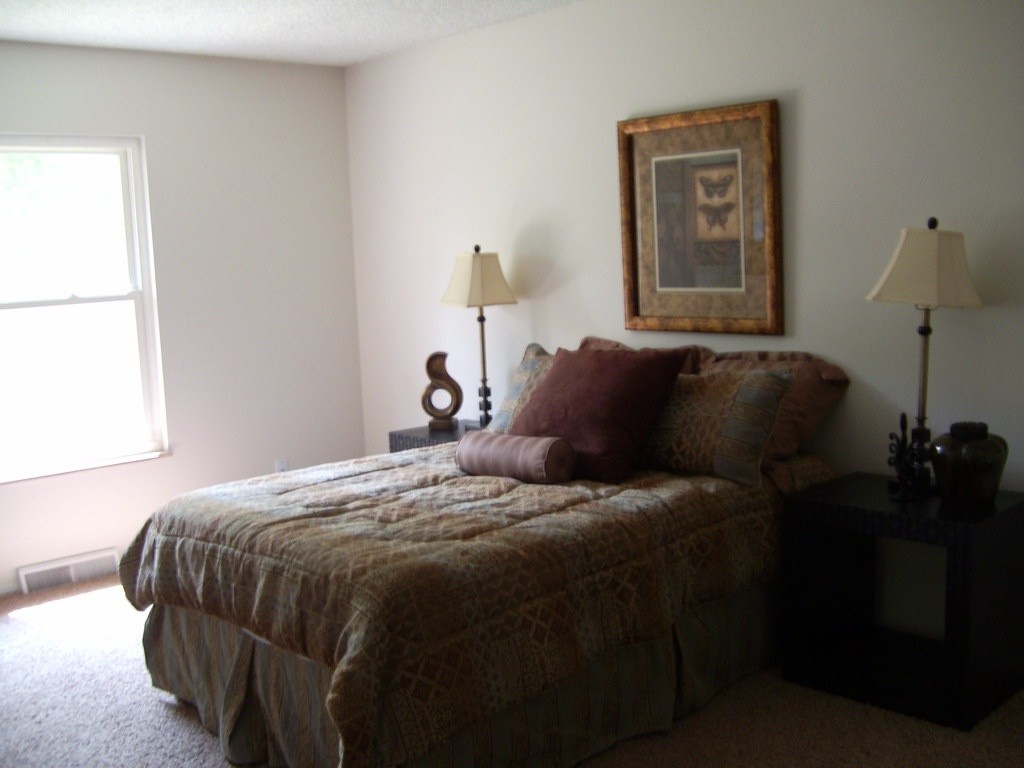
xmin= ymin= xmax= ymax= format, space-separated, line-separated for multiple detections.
xmin=616 ymin=99 xmax=784 ymax=337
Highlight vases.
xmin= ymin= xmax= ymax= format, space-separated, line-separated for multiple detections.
xmin=927 ymin=419 xmax=1009 ymax=516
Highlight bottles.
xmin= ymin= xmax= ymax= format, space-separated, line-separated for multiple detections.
xmin=928 ymin=422 xmax=1009 ymax=508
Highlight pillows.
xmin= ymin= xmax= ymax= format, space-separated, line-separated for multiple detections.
xmin=508 ymin=347 xmax=691 ymax=486
xmin=456 ymin=429 xmax=577 ymax=485
xmin=704 ymin=349 xmax=851 ymax=478
xmin=633 ymin=369 xmax=794 ymax=494
xmin=480 ymin=344 xmax=557 ymax=431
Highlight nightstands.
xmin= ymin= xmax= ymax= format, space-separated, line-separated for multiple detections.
xmin=779 ymin=469 xmax=1024 ymax=732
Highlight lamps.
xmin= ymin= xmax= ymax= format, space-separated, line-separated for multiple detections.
xmin=861 ymin=216 xmax=985 ymax=499
xmin=439 ymin=243 xmax=518 ymax=433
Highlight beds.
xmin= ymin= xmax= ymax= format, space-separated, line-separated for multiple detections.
xmin=118 ymin=453 xmax=847 ymax=768
